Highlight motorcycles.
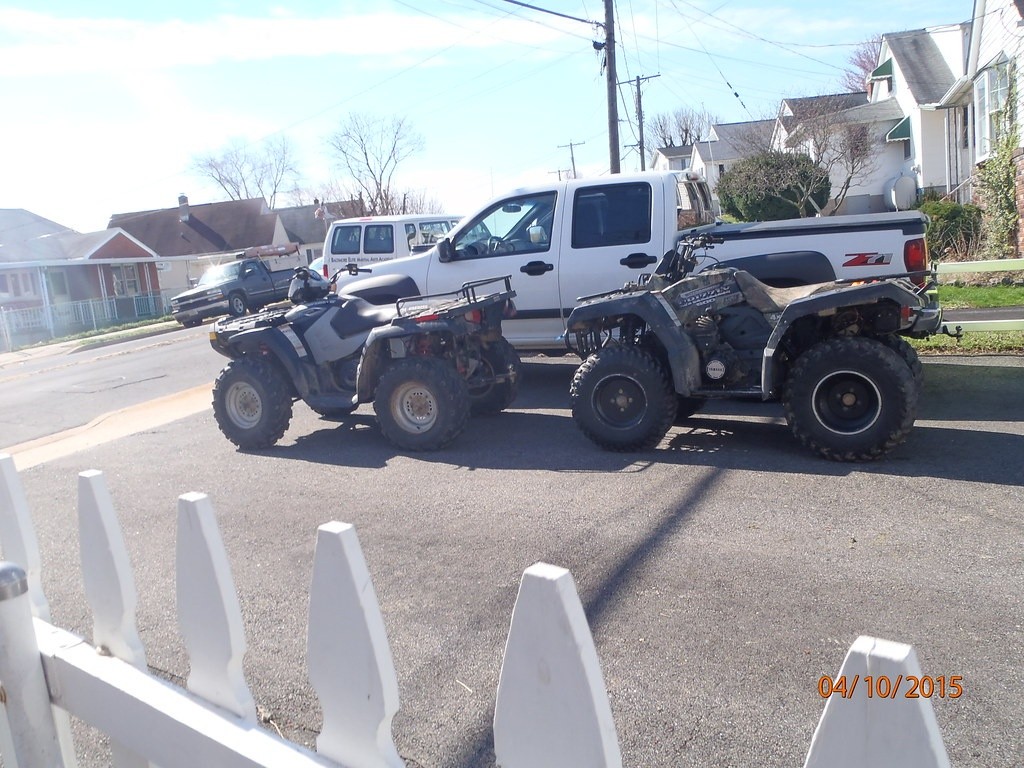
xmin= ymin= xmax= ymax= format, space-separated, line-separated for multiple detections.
xmin=564 ymin=229 xmax=943 ymax=462
xmin=208 ymin=263 xmax=523 ymax=452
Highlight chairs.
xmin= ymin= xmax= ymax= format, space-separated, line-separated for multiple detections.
xmin=575 ymin=204 xmax=600 ymax=247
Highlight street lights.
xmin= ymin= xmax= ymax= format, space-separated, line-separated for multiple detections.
xmin=320 ymin=197 xmax=328 ymax=233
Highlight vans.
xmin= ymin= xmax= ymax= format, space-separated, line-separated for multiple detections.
xmin=322 ymin=214 xmax=493 ymax=284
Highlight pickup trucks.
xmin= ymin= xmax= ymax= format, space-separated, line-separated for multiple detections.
xmin=170 ymin=253 xmax=296 ymax=328
xmin=330 ymin=170 xmax=963 ymax=358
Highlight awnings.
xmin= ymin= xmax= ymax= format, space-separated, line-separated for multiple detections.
xmin=866 ymin=57 xmax=891 ymax=83
xmin=882 ymin=115 xmax=910 ymax=142
